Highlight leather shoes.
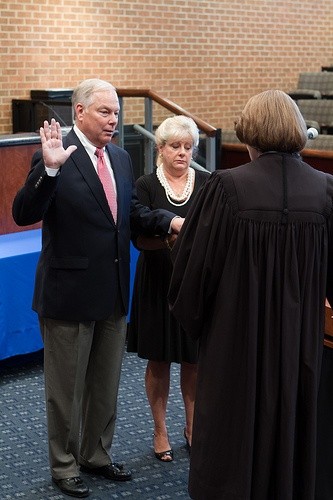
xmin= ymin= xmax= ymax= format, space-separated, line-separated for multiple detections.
xmin=80 ymin=463 xmax=133 ymax=482
xmin=52 ymin=476 xmax=90 ymax=498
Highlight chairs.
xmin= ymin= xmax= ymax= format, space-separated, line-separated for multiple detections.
xmin=217 ymin=64 xmax=333 ymax=149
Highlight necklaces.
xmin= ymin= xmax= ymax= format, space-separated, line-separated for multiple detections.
xmin=156 ymin=163 xmax=195 ymax=207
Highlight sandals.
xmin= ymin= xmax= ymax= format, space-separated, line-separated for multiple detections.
xmin=154 ymin=449 xmax=174 ymax=462
xmin=184 ymin=428 xmax=191 ymax=452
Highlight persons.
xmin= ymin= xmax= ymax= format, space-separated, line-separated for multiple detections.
xmin=166 ymin=90 xmax=333 ymax=500
xmin=130 ymin=115 xmax=211 ymax=462
xmin=10 ymin=80 xmax=134 ymax=498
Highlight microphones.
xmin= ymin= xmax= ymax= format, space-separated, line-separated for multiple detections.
xmin=306 ymin=128 xmax=318 ymax=140
xmin=39 ymin=101 xmax=66 ymax=127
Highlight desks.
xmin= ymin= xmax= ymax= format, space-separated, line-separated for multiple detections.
xmin=1 ymin=228 xmax=142 ymax=363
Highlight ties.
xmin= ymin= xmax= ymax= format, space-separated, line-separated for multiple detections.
xmin=94 ymin=148 xmax=117 ymax=225
